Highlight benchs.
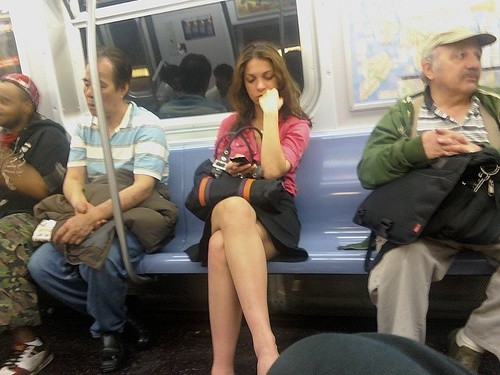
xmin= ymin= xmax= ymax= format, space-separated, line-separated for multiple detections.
xmin=136 ymin=132 xmax=497 ymax=276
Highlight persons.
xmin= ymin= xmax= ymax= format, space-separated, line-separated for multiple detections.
xmin=205 ymin=62 xmax=236 ymax=112
xmin=27 ymin=45 xmax=170 ymax=369
xmin=356 ymin=27 xmax=500 ymax=373
xmin=200 ymin=40 xmax=311 ymax=373
xmin=157 ymin=51 xmax=227 ymax=116
xmin=1 ymin=72 xmax=71 ymax=375
xmin=152 ymin=63 xmax=182 ymax=108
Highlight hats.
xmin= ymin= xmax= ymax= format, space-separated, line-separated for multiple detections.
xmin=419 ymin=26 xmax=497 ymax=62
xmin=0 ymin=73 xmax=39 ymax=110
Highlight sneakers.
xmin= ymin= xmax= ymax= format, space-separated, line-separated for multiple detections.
xmin=0 ymin=339 xmax=54 ymax=375
xmin=448 ymin=328 xmax=482 ymax=374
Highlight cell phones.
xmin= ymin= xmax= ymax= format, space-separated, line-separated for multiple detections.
xmin=230 ymin=157 xmax=251 ymax=166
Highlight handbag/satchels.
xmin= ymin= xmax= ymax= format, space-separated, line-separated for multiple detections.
xmin=184 ymin=155 xmax=285 ymax=221
xmin=352 ymin=145 xmax=499 ymax=245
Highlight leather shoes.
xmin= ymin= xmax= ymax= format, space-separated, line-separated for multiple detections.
xmin=127 ymin=311 xmax=155 ymax=351
xmin=97 ymin=330 xmax=124 ymax=374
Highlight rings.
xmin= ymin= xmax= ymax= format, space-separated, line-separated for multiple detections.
xmin=236 ymin=171 xmax=244 ymax=178
